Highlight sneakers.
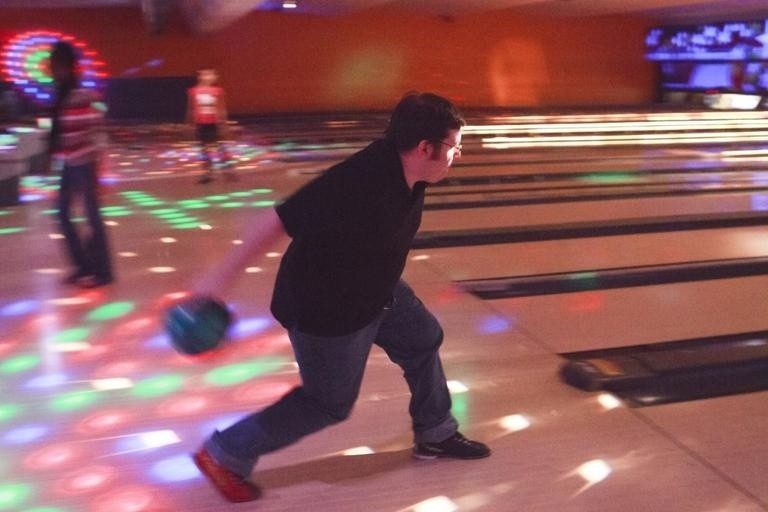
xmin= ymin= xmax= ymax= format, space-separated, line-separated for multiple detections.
xmin=190 ymin=446 xmax=263 ymax=503
xmin=410 ymin=431 xmax=490 ymax=462
xmin=58 ymin=268 xmax=113 ymax=289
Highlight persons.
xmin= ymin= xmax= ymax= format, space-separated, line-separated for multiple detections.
xmin=39 ymin=41 xmax=116 ymax=289
xmin=183 ymin=68 xmax=239 ymax=186
xmin=183 ymin=89 xmax=492 ymax=503
